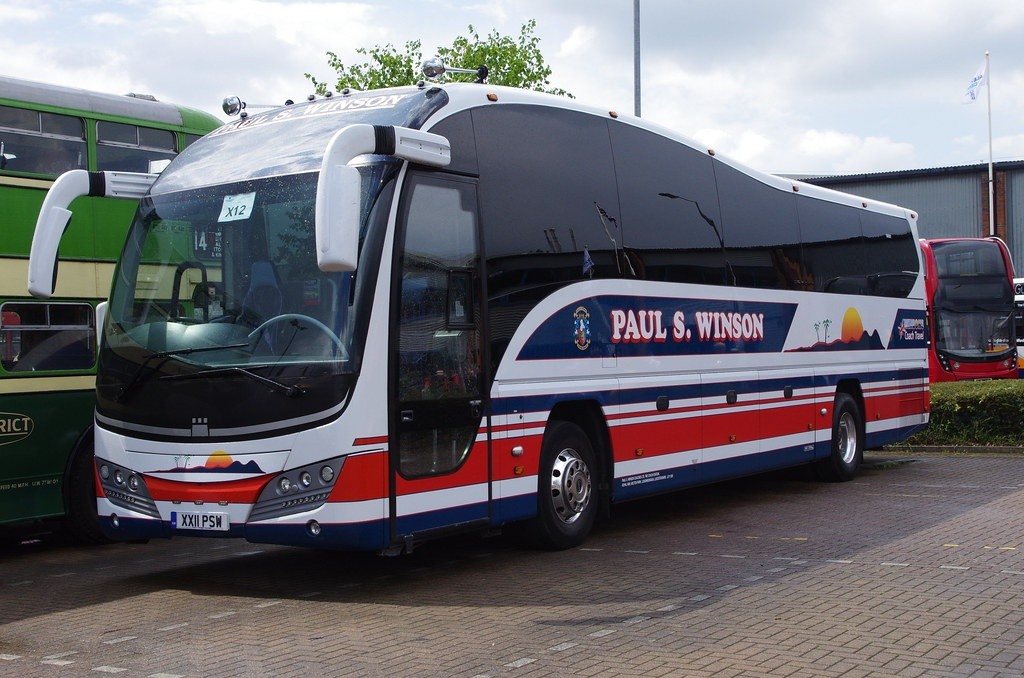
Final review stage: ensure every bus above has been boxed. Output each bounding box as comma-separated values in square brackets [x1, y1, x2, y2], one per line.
[24, 63, 932, 558]
[0, 76, 228, 546]
[919, 236, 1024, 383]
